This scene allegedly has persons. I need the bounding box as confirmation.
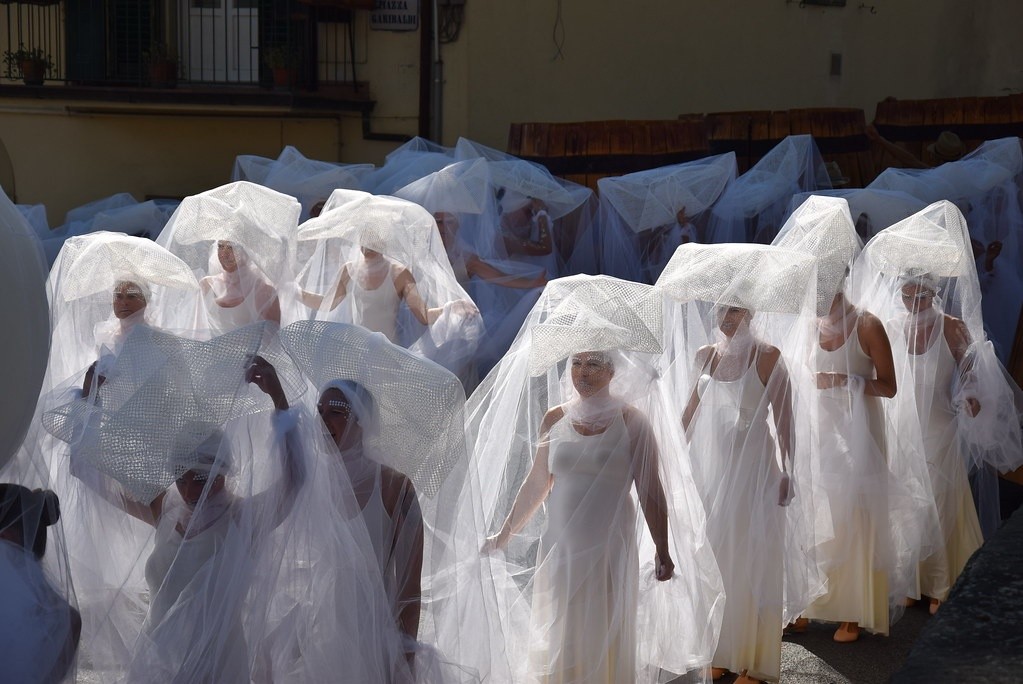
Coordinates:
[0,133,1023,684]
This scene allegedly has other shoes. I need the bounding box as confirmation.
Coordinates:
[698,667,729,679]
[833,622,859,642]
[731,674,761,684]
[928,598,941,614]
[785,617,809,633]
[895,596,916,607]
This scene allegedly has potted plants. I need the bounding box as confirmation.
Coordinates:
[2,42,55,85]
[142,40,186,88]
[265,44,306,86]
